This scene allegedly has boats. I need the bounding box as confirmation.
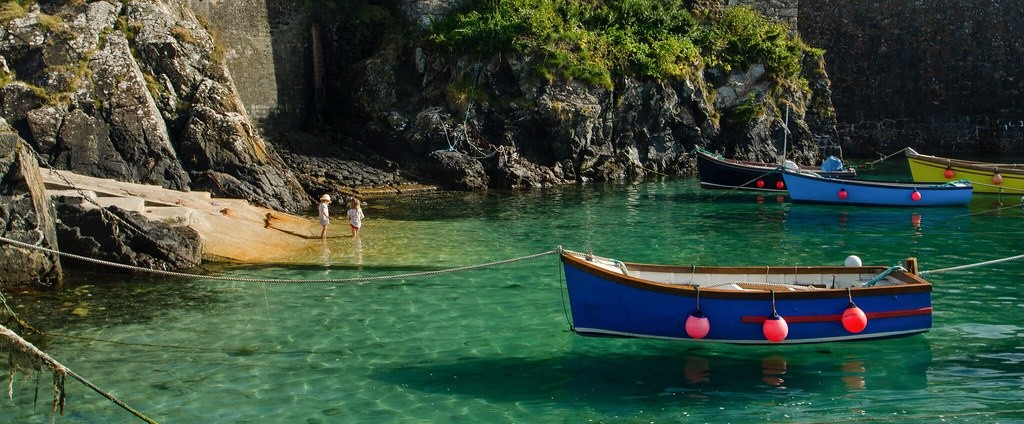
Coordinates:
[557,244,935,345]
[903,145,1024,196]
[779,160,973,208]
[694,102,857,207]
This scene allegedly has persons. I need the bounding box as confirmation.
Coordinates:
[319,194,332,239]
[347,198,365,239]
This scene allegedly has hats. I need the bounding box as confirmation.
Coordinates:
[320,194,331,203]
